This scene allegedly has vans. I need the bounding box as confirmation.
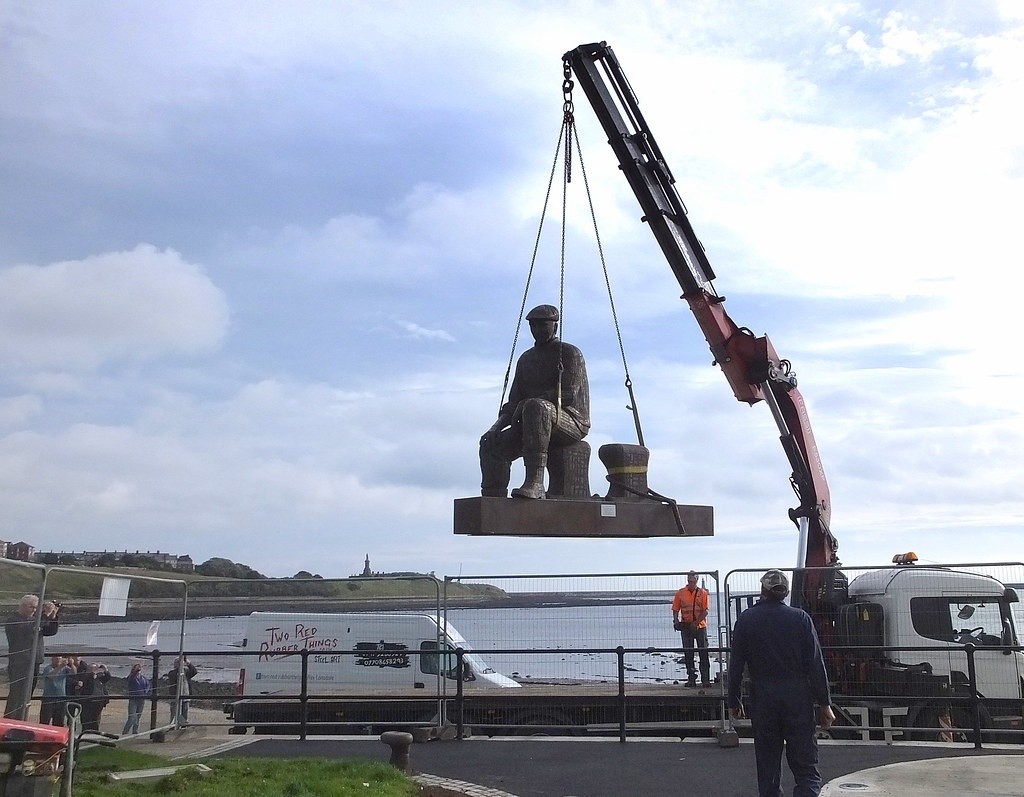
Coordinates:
[237,609,523,695]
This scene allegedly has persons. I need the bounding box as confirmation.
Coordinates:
[122,664,150,734]
[168,657,198,730]
[3,595,59,721]
[66,656,111,734]
[479,305,591,499]
[727,570,836,797]
[671,570,710,687]
[39,656,77,727]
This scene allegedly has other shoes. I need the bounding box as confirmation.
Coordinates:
[703,681,711,687]
[684,679,696,687]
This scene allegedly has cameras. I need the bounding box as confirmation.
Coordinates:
[51,599,63,606]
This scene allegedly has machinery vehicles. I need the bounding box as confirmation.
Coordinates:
[221,40,1023,747]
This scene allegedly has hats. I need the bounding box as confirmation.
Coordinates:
[760,569,789,597]
